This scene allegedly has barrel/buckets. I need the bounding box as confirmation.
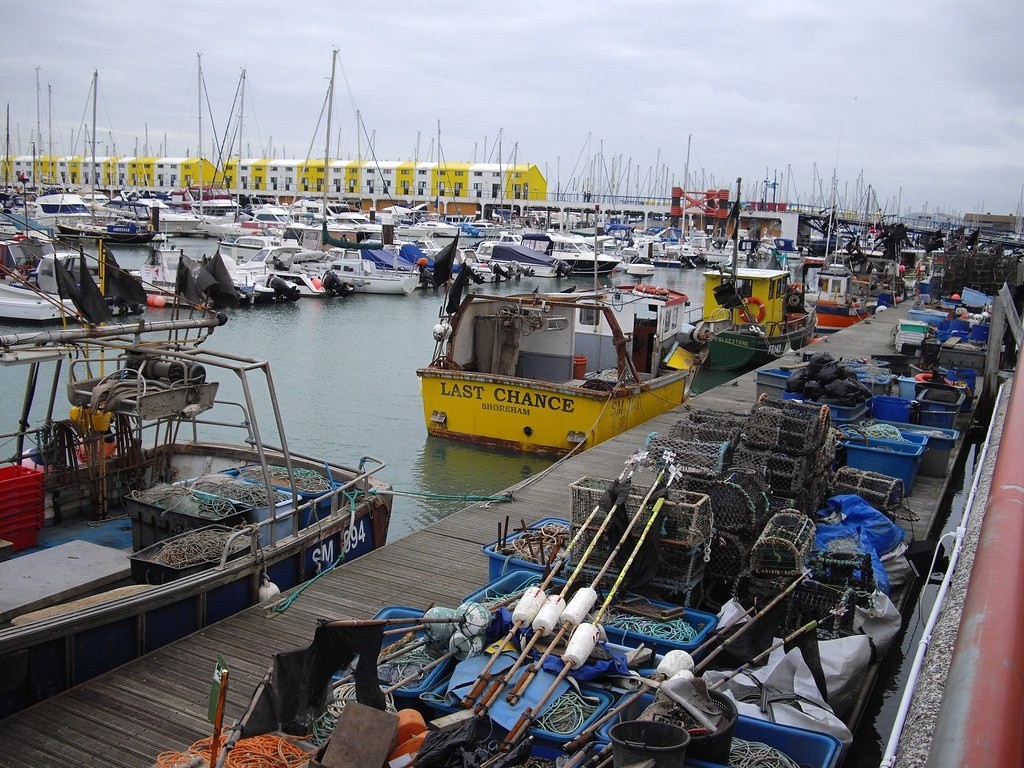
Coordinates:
[939,294,963,309]
[803,352,814,363]
[897,376,917,401]
[947,369,977,412]
[970,325,989,344]
[786,312,805,330]
[782,389,803,403]
[872,395,911,423]
[573,356,586,380]
[949,318,970,332]
[609,719,691,768]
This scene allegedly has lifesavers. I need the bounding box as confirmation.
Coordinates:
[793,283,802,293]
[914,372,953,387]
[636,284,670,295]
[739,297,766,324]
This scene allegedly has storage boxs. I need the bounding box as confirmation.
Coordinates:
[122,492,254,552]
[333,287,993,768]
[218,464,344,531]
[171,475,303,548]
[0,464,46,551]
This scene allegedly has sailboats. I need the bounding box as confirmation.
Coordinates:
[0,44,814,367]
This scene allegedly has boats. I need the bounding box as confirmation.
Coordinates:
[0,214,395,721]
[415,228,733,461]
[684,177,818,373]
[790,177,946,333]
